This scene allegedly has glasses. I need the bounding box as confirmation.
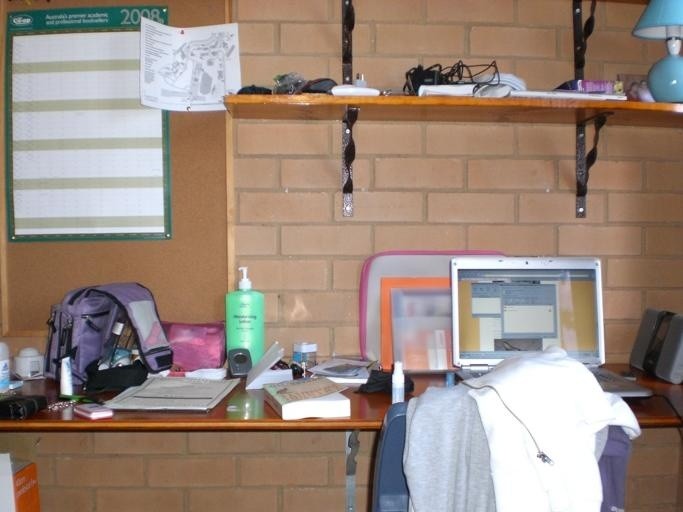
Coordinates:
[301,77,335,93]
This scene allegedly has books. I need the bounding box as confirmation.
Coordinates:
[266,373,356,421]
[311,358,376,385]
[103,375,240,412]
[245,341,293,390]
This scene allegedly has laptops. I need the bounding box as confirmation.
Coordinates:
[451,256,653,398]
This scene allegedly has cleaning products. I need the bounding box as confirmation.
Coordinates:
[391,360,405,403]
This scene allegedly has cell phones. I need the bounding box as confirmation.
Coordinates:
[74,404,113,420]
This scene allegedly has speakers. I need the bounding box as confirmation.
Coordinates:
[655,313,683,385]
[629,308,674,371]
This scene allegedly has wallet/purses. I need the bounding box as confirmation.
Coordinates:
[1,395,48,420]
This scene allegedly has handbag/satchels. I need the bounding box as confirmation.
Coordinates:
[167,321,226,370]
[44,286,126,387]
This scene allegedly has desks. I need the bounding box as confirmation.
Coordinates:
[0,363,681,512]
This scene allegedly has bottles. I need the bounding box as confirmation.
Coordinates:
[0,341,12,394]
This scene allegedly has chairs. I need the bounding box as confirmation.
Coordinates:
[373,401,631,512]
